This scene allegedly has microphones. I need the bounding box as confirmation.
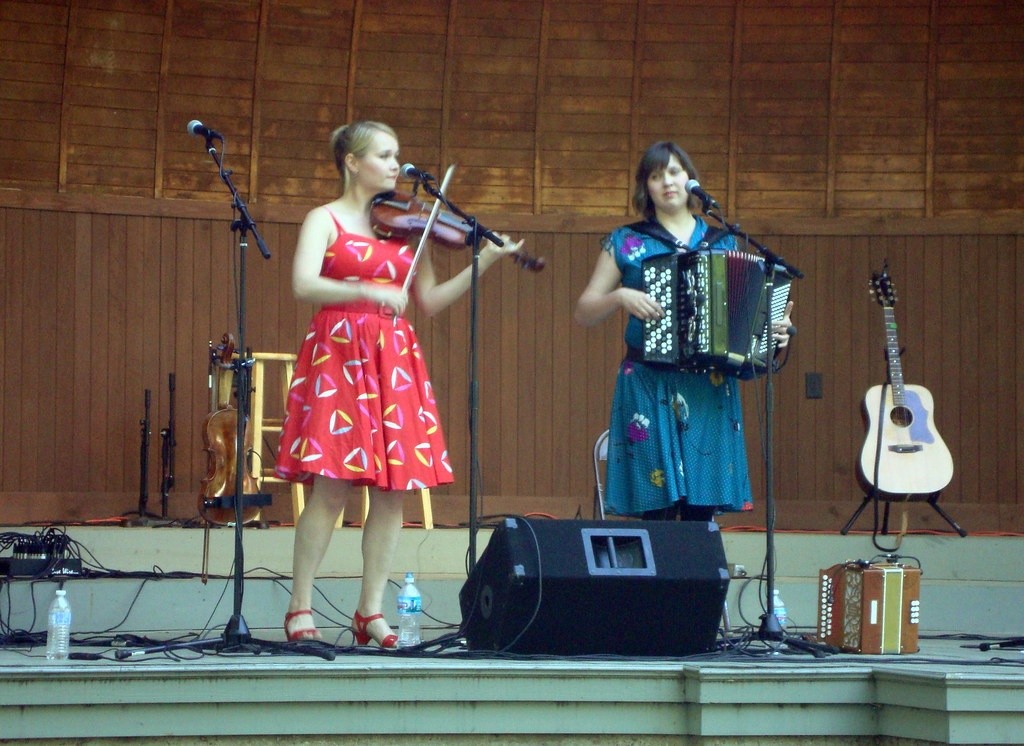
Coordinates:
[187,119,223,139]
[400,163,435,180]
[684,180,720,210]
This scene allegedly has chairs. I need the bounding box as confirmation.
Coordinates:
[593,429,731,634]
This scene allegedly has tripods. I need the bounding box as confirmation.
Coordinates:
[841,345,967,538]
[114,136,337,662]
[701,204,844,658]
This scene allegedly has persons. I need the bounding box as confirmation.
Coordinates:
[575,141,794,523]
[274,122,527,650]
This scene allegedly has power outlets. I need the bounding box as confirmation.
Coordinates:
[806,373,823,399]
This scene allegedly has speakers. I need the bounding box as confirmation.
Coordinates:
[459,520,731,660]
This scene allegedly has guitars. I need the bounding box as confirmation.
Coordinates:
[856,255,954,494]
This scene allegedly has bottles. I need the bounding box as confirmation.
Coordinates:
[765,590,786,655]
[46,591,71,660]
[398,574,422,649]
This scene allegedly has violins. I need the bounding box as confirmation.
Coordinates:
[199,331,261,526]
[371,191,545,273]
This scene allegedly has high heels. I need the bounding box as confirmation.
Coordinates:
[284,609,321,642]
[352,610,398,648]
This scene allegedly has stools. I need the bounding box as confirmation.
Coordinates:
[217,352,433,530]
